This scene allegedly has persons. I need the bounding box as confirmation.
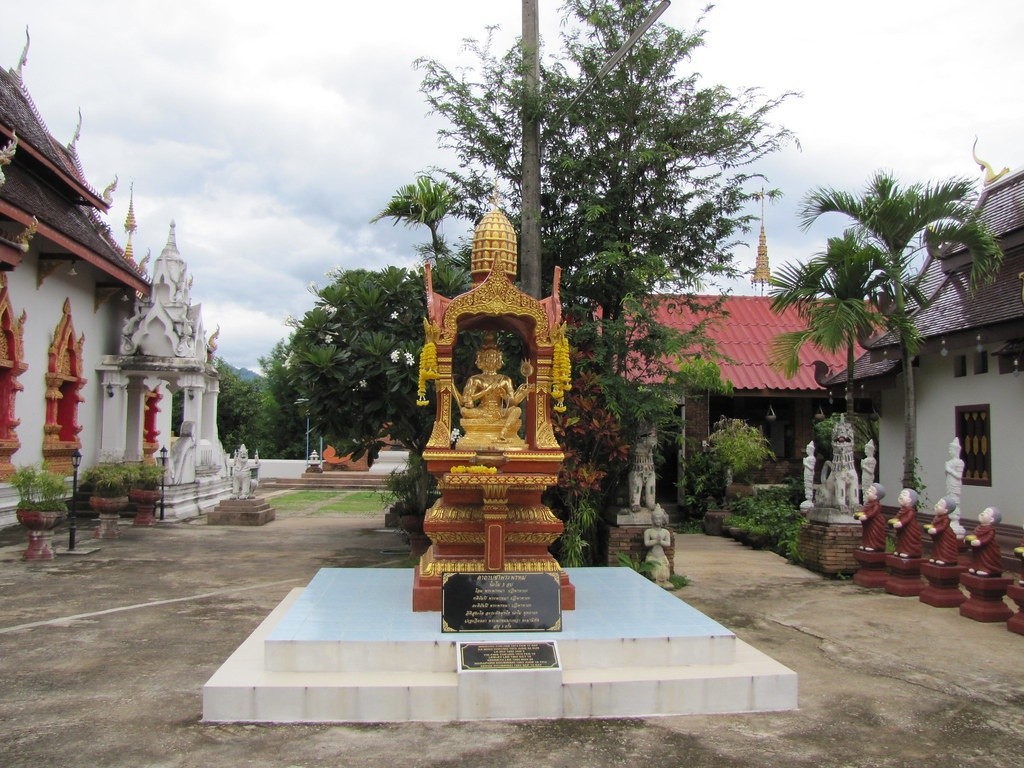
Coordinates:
[928,496,959,564]
[644,503,670,582]
[893,488,922,557]
[964,507,1003,575]
[452,335,535,441]
[628,427,657,512]
[803,440,816,500]
[853,483,886,550]
[945,437,964,523]
[860,438,876,505]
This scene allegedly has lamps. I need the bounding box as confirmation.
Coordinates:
[187,385,195,400]
[160,446,168,459]
[120,288,130,303]
[106,382,113,397]
[72,448,81,466]
[67,260,78,276]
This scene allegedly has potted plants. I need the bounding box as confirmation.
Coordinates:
[2,456,76,565]
[79,449,140,541]
[127,455,167,526]
[702,502,771,548]
[379,461,434,557]
